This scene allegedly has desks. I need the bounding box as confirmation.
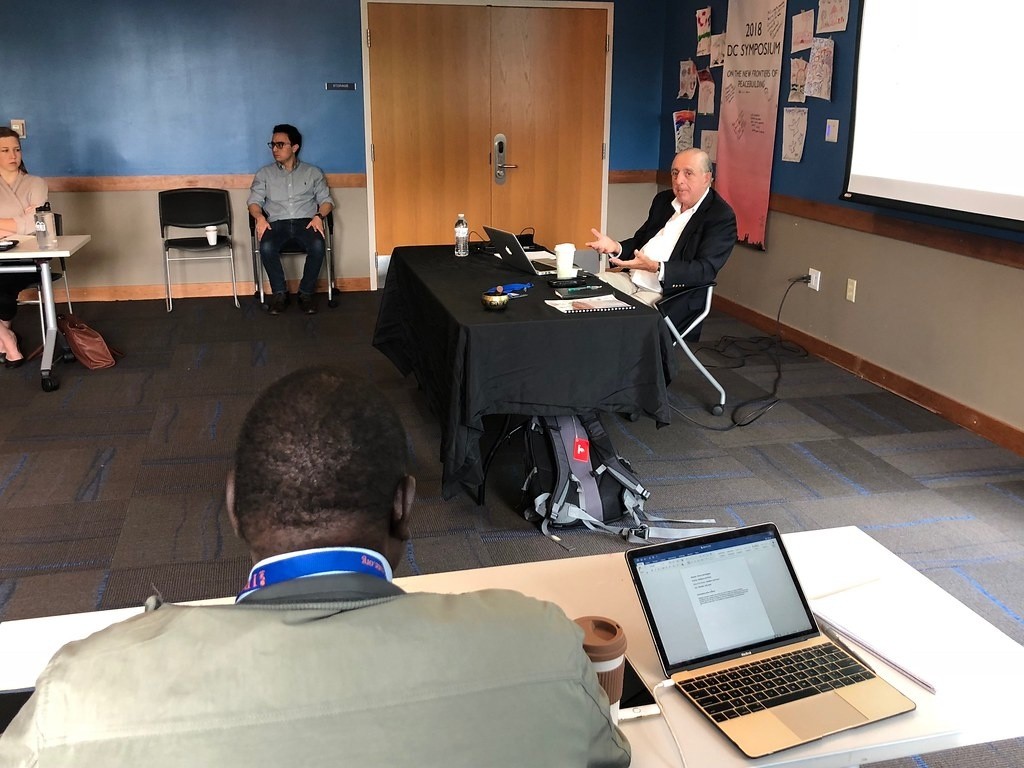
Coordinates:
[372,240,676,506]
[0,524,1024,767]
[0,235,93,392]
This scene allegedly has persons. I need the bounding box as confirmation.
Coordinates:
[248,124,336,317]
[0,367,632,768]
[0,126,49,366]
[584,147,737,329]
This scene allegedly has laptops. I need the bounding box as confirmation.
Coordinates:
[483,226,582,276]
[624,522,917,758]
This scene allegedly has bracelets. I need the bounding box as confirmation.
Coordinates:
[314,212,325,220]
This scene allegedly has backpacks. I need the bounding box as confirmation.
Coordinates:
[496,412,651,550]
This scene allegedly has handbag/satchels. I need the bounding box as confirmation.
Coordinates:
[57,314,126,370]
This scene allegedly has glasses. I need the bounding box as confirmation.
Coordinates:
[267,142,291,149]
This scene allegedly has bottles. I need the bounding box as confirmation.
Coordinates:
[34,202,58,251]
[454,213,470,256]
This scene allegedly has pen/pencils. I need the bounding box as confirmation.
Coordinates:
[567,286,602,292]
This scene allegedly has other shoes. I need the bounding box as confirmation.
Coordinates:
[294,291,316,315]
[0,353,6,364]
[268,292,288,315]
[4,330,25,370]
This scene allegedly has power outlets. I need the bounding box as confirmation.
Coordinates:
[808,267,820,291]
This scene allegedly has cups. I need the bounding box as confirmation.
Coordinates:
[572,616,628,727]
[555,243,576,280]
[205,225,217,245]
[481,292,509,311]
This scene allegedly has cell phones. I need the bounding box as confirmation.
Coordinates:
[619,654,662,721]
[547,279,586,288]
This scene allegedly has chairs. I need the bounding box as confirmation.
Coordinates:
[653,281,726,415]
[25,214,74,348]
[249,204,341,312]
[158,189,241,313]
